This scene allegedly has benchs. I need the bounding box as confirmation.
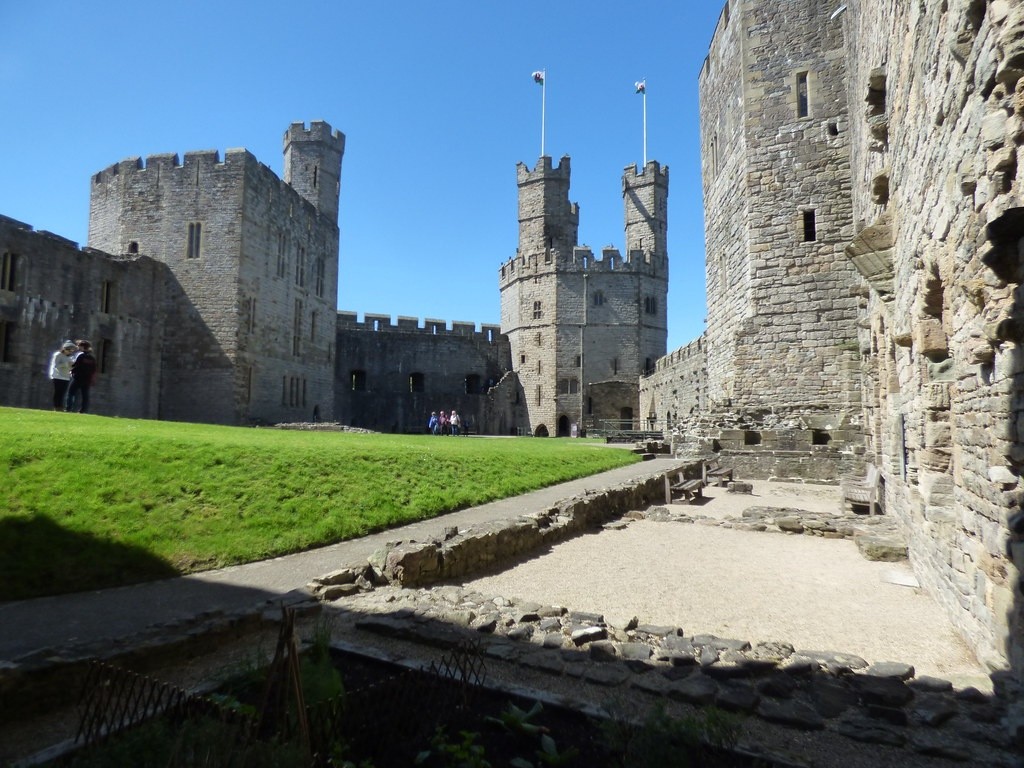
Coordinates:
[840,463,882,516]
[664,465,702,504]
[702,455,732,486]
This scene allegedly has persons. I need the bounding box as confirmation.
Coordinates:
[63,339,96,413]
[429,410,470,437]
[49,340,76,411]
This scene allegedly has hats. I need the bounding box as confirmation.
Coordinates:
[63,340,75,350]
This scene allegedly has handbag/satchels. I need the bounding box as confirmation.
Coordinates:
[458,423,461,428]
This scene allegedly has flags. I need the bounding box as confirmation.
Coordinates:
[635,81,645,94]
[532,71,544,86]
